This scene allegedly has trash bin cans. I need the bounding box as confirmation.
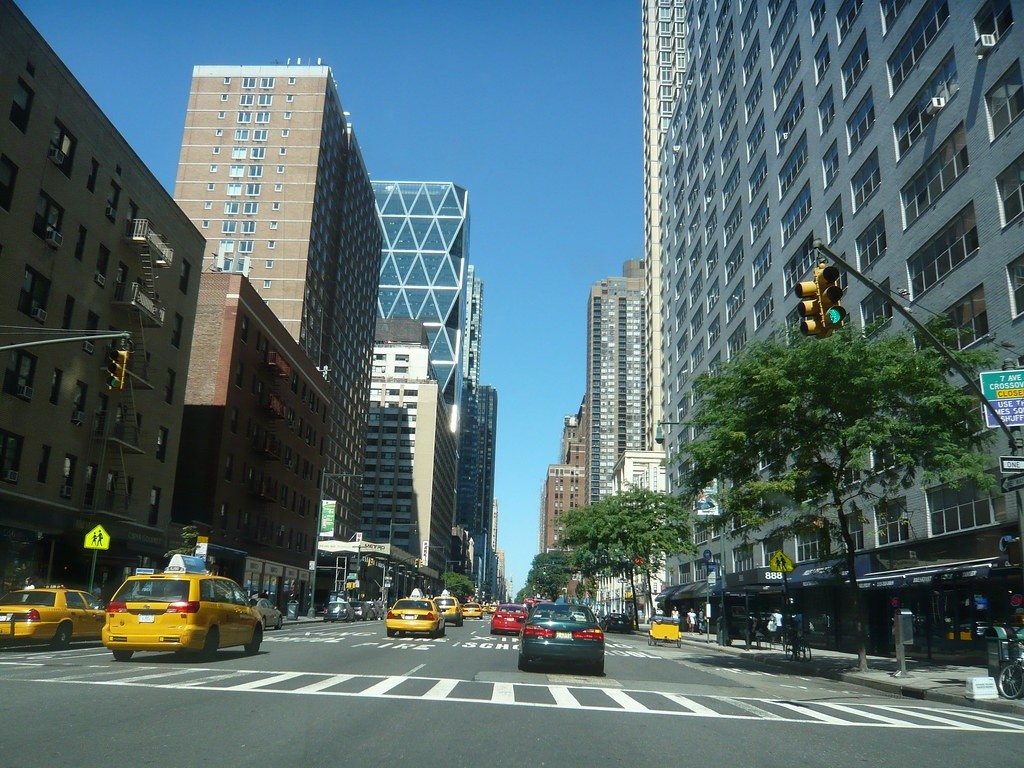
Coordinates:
[287,600,300,621]
[717,616,729,642]
[895,608,914,645]
[985,626,1024,696]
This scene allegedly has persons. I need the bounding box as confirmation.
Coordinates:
[377,597,385,617]
[92,580,102,599]
[288,593,296,600]
[671,606,680,620]
[595,609,601,623]
[766,608,784,645]
[696,611,706,634]
[687,607,697,633]
[268,591,276,605]
[260,589,268,598]
[20,576,35,602]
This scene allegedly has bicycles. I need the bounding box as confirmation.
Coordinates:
[997,637,1024,700]
[784,624,811,662]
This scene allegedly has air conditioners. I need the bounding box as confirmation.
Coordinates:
[927,96,944,114]
[975,34,994,54]
[61,486,72,498]
[781,132,788,143]
[672,145,679,154]
[106,207,118,221]
[47,149,65,164]
[3,470,18,482]
[83,341,94,353]
[72,411,86,422]
[31,307,47,322]
[17,385,32,399]
[45,230,64,246]
[94,274,106,286]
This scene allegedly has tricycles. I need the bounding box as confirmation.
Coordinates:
[647,615,682,648]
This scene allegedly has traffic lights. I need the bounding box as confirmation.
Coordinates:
[794,280,829,339]
[814,265,847,330]
[419,559,424,569]
[106,350,130,389]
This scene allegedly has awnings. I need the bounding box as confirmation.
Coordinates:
[856,555,1001,590]
[711,581,753,597]
[655,577,720,602]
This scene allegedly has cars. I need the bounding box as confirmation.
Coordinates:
[481,598,546,614]
[101,553,265,663]
[323,600,385,623]
[461,603,483,620]
[385,588,445,639]
[515,601,605,676]
[598,612,632,635]
[0,584,109,648]
[432,589,464,627]
[490,603,528,636]
[249,597,283,631]
